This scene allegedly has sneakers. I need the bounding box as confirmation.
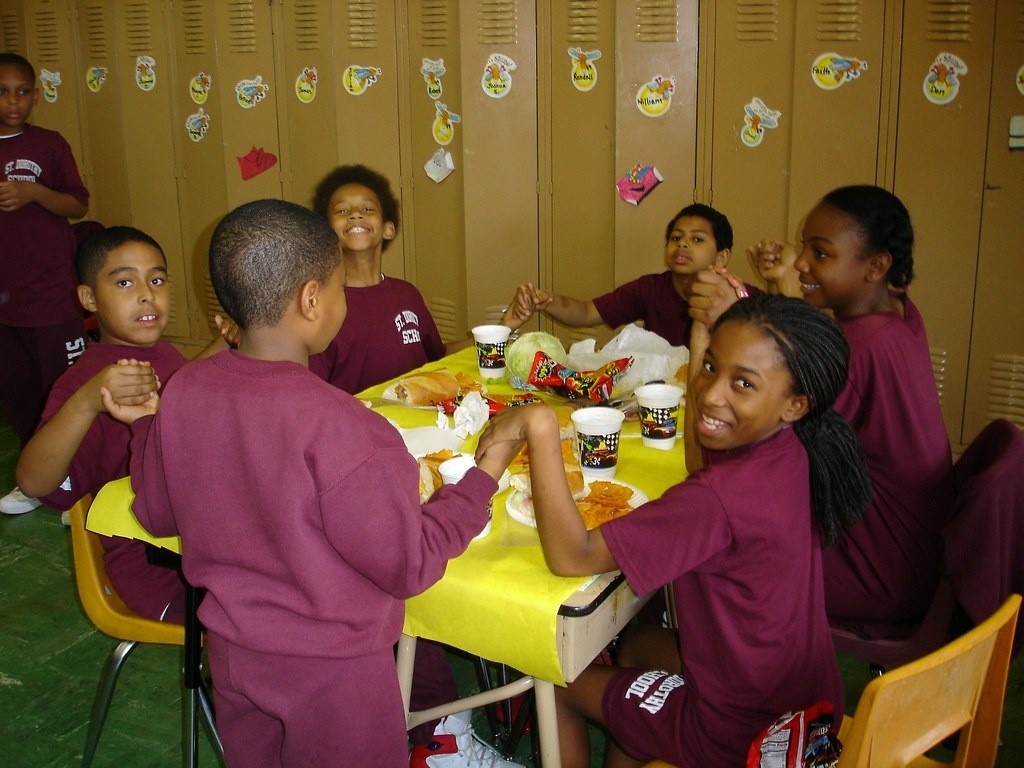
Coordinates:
[0,486,42,514]
[410,709,525,768]
[61,510,71,527]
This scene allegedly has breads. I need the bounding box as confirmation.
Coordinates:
[417,448,463,502]
[674,363,688,384]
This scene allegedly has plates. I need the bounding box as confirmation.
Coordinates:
[504,474,650,529]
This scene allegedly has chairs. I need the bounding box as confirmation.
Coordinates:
[61,492,227,768]
[817,416,1024,768]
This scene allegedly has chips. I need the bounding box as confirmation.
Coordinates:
[577,480,636,530]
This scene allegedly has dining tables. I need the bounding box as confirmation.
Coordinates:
[86,332,691,768]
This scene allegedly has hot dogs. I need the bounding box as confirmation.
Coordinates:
[395,368,483,405]
[509,463,585,498]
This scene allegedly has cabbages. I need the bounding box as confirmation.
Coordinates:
[504,331,569,387]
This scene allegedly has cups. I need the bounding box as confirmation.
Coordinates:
[472,325,511,379]
[635,385,684,451]
[571,407,626,478]
[438,456,493,540]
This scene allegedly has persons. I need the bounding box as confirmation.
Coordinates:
[510,202,766,348]
[686,184,954,625]
[16,224,455,747]
[0,53,90,515]
[99,199,525,768]
[221,166,553,395]
[476,293,844,768]
